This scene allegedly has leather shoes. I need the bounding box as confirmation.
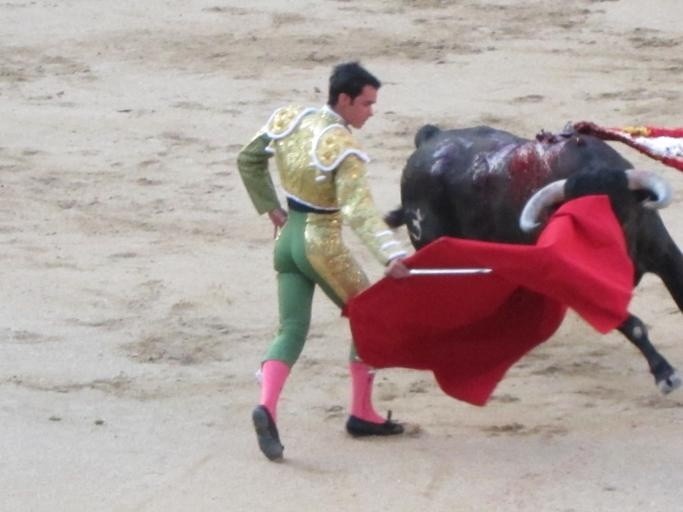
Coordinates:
[346,414,404,436]
[252,405,283,464]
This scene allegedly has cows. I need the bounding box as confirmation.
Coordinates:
[382,123,682,395]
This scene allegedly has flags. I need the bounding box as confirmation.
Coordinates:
[333,193,634,408]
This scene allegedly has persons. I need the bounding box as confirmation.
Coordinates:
[235,60,406,462]
